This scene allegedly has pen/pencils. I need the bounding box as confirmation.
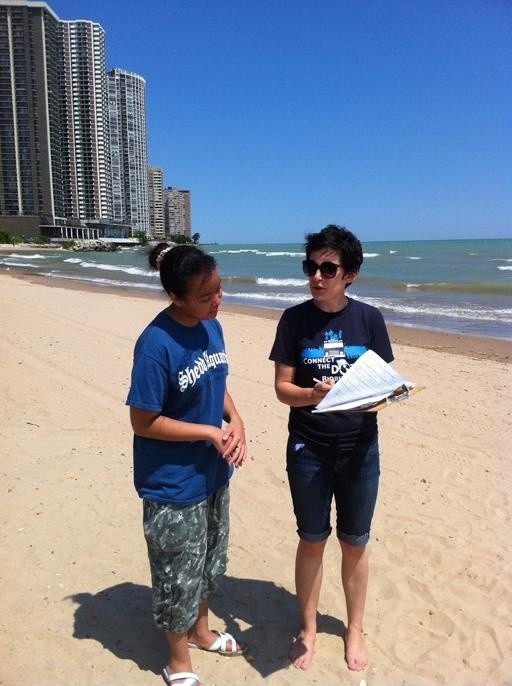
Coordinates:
[313,378,333,389]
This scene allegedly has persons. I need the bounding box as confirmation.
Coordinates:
[126,243,247,686]
[268,224,395,672]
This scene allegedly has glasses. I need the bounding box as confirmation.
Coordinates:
[302,260,344,279]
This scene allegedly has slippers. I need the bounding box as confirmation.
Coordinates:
[187,629,249,657]
[163,666,201,685]
